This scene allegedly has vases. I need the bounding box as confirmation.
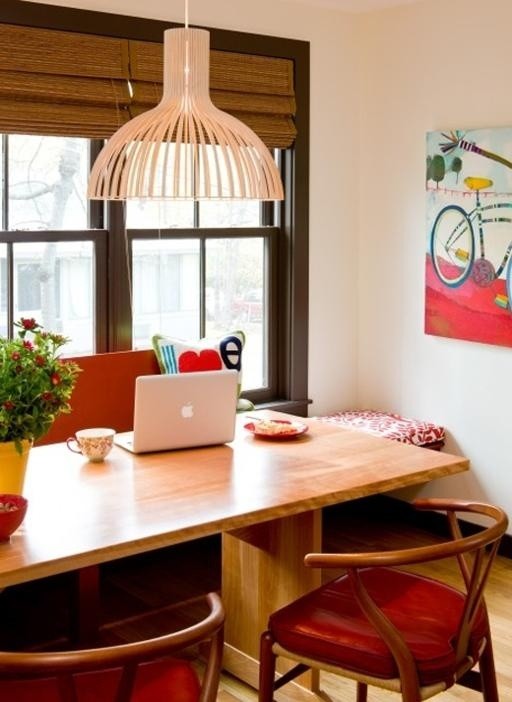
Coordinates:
[0,436,33,498]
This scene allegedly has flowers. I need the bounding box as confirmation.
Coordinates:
[0,318,86,445]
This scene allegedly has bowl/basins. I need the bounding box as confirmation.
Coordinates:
[0,493,28,544]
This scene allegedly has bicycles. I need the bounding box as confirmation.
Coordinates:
[431,177,512,311]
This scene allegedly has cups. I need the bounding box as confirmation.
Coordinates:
[67,429,115,464]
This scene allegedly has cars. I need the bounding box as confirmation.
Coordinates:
[228,294,262,320]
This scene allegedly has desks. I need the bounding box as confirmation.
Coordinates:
[307,409,447,451]
[2,408,470,702]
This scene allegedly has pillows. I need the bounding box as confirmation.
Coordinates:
[151,329,256,412]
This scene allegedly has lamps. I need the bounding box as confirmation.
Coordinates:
[86,0,288,202]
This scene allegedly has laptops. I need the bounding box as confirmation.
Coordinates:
[111,369,238,453]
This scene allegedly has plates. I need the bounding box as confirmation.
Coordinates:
[244,419,313,441]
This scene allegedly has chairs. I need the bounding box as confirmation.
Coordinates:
[0,591,225,700]
[255,496,509,702]
[36,348,165,448]
